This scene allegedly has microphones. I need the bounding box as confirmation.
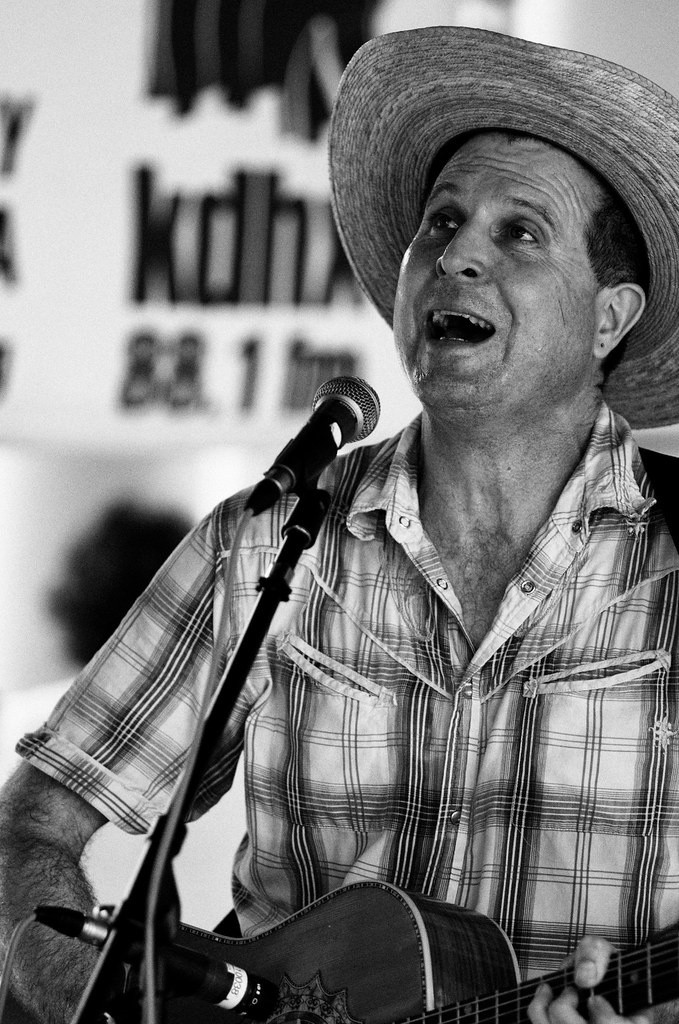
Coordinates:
[244,376,381,517]
[33,905,277,1020]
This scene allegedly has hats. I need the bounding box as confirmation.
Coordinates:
[326,26,679,429]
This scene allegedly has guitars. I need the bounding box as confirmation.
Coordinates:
[163,880,679,1024]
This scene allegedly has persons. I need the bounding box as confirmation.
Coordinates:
[0,26,679,1024]
[1,502,244,933]
